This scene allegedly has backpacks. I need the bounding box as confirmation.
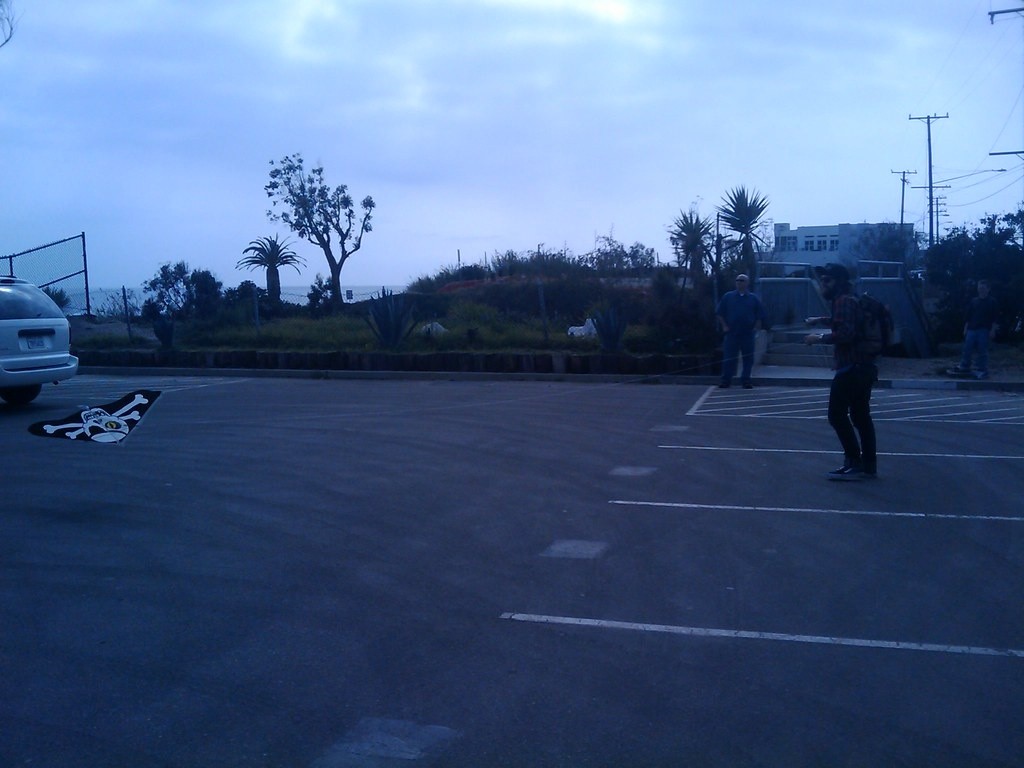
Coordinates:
[854,293,893,355]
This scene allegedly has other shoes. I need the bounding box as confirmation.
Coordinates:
[948,368,969,374]
[740,379,752,388]
[718,384,730,388]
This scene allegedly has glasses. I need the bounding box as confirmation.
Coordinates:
[735,279,746,282]
[818,277,837,283]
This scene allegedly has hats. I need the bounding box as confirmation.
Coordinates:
[815,263,849,283]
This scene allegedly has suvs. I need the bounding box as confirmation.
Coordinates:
[0,274,80,404]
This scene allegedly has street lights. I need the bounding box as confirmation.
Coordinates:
[928,169,1007,249]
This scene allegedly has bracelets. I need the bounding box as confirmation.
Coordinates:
[819,334,823,343]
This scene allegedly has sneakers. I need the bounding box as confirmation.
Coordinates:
[859,465,877,477]
[825,465,864,481]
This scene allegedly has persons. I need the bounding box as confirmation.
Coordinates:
[715,274,763,388]
[947,278,998,379]
[802,263,883,480]
